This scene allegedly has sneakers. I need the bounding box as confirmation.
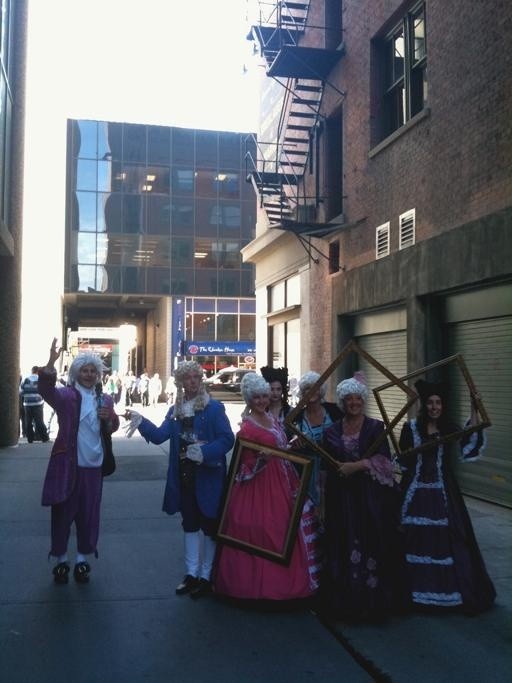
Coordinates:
[190,577,209,597]
[52,563,69,583]
[73,561,90,583]
[175,574,198,595]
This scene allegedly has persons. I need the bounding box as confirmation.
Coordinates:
[116,360,236,599]
[322,378,406,622]
[138,373,149,408]
[211,372,322,602]
[294,370,345,507]
[125,370,137,407]
[36,337,121,585]
[259,365,296,444]
[21,365,51,444]
[106,369,118,401]
[395,379,497,616]
[148,373,163,406]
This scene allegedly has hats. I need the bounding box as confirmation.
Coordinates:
[415,373,444,396]
[260,367,282,381]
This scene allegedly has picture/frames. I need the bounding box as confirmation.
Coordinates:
[212,435,315,567]
[372,352,492,462]
[282,341,418,482]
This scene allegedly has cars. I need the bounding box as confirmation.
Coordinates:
[202,367,256,402]
